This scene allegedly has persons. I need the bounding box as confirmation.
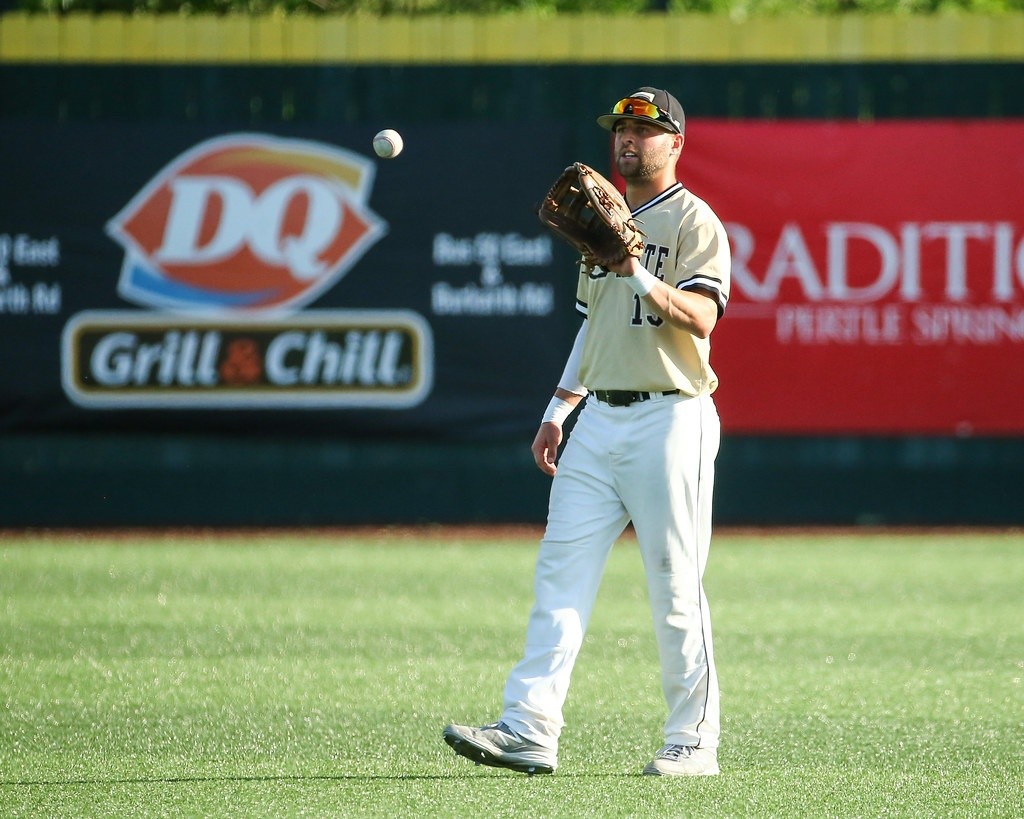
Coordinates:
[443,87,731,779]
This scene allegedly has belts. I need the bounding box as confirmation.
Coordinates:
[587,389,681,407]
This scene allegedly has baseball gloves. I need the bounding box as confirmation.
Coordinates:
[538,162,645,273]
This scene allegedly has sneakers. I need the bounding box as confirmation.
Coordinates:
[643,744,719,776]
[443,721,557,777]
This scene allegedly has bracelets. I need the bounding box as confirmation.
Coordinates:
[541,395,575,427]
[621,265,663,299]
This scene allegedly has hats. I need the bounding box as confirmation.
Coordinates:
[597,87,685,136]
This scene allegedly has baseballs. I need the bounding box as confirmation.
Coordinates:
[372,129,405,159]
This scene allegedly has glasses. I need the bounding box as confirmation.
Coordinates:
[611,98,680,134]
